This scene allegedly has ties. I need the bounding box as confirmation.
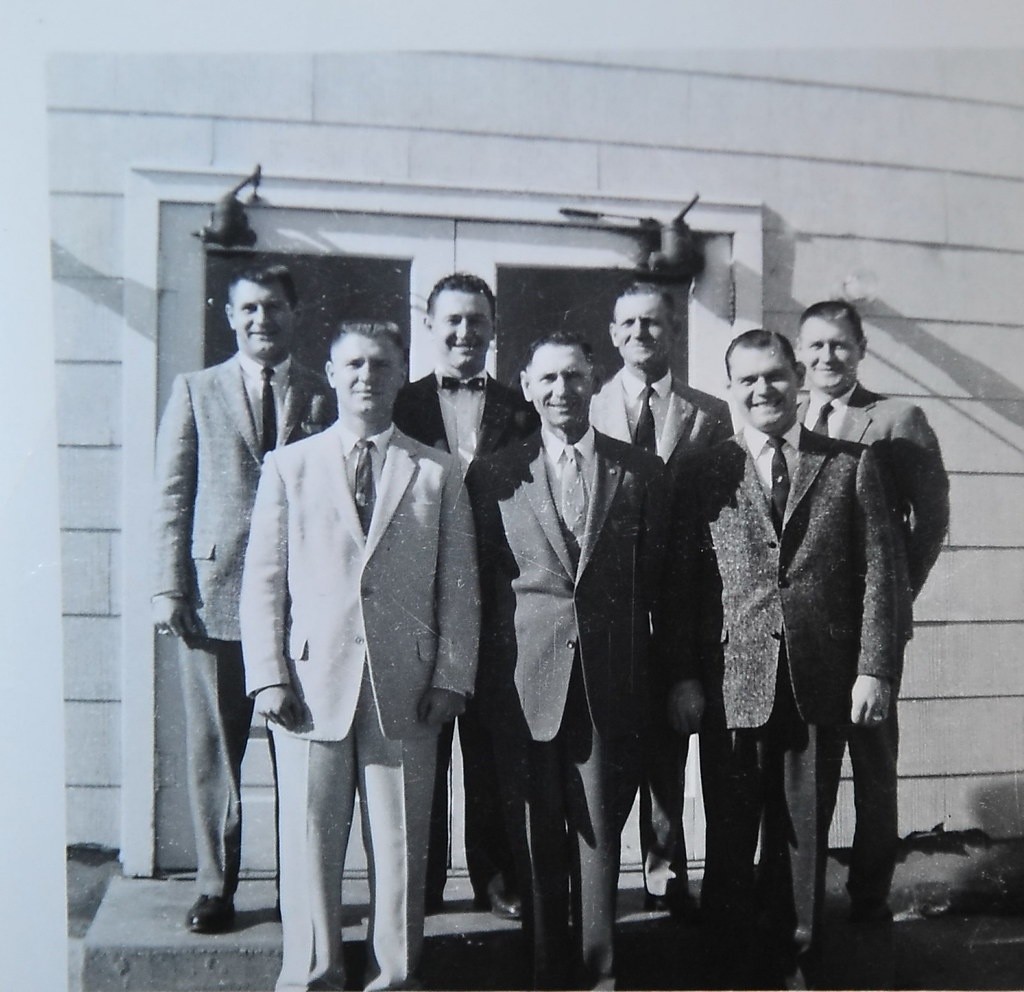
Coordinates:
[632,386,656,455]
[562,445,588,575]
[766,436,790,540]
[812,402,835,436]
[262,368,279,454]
[353,440,376,538]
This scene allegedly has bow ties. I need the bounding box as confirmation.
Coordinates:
[440,376,486,390]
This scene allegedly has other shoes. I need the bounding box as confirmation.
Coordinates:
[646,891,703,921]
[853,895,893,925]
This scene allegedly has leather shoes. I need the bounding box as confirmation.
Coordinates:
[187,893,238,931]
[474,884,522,918]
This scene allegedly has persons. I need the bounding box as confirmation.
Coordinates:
[794,302,950,944]
[461,331,678,991]
[393,274,542,918]
[150,261,339,931]
[239,320,481,992]
[676,333,900,991]
[589,283,735,910]
